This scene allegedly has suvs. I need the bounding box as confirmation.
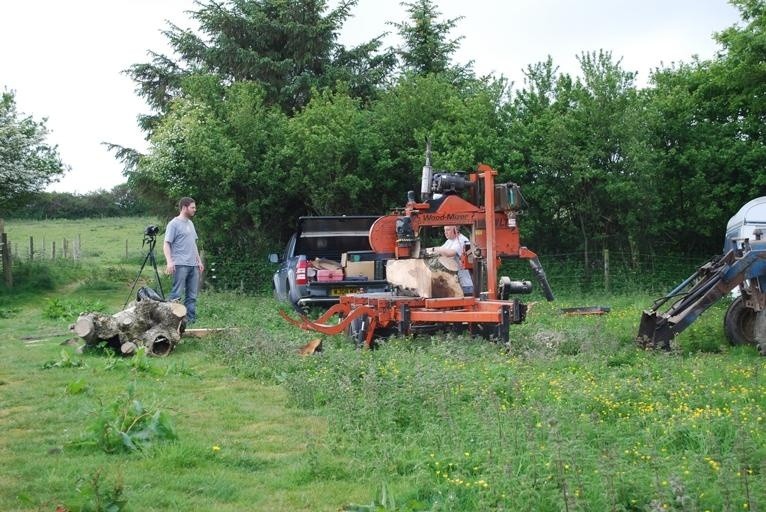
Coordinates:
[267,213,398,315]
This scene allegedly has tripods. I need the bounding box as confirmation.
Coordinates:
[123,238,170,311]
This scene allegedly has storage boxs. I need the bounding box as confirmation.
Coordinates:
[346,261,375,281]
[317,270,343,281]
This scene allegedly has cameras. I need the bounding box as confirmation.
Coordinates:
[145,226,158,236]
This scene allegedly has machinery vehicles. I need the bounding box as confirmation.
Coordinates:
[636,195,766,353]
[276,135,610,352]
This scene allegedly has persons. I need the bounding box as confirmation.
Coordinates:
[163,197,204,323]
[434,225,473,294]
